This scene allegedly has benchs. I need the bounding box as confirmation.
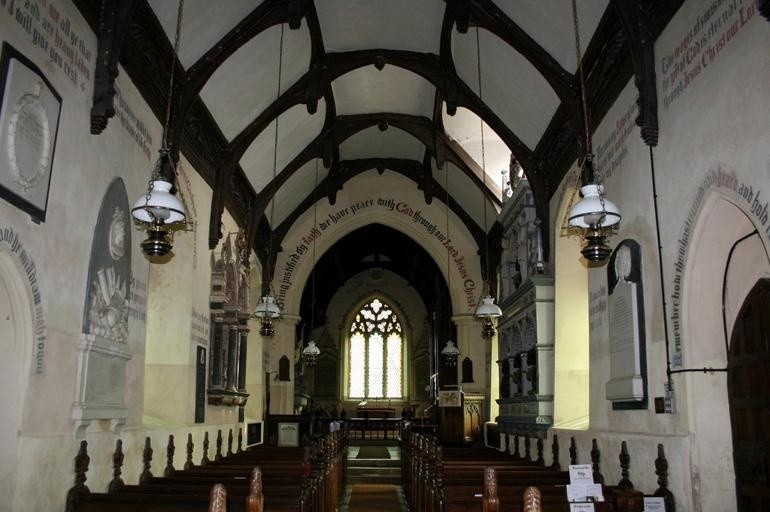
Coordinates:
[65,430,346,512]
[402,429,676,512]
